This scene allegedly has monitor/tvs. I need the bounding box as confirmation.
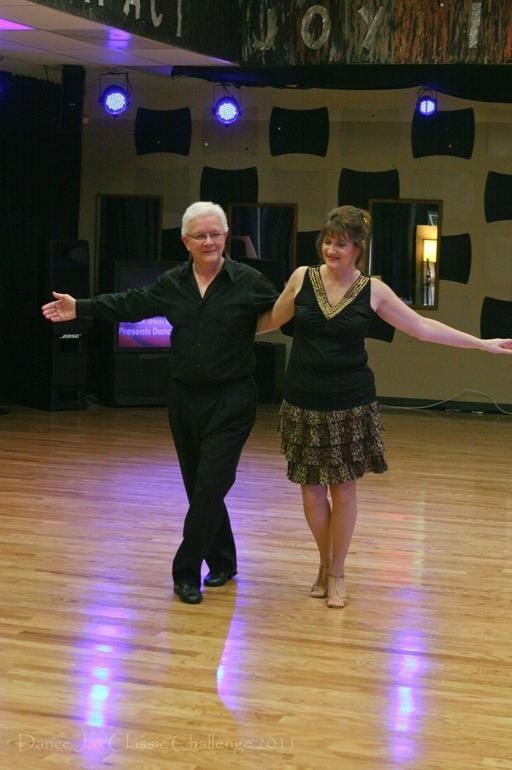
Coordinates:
[112,316,173,353]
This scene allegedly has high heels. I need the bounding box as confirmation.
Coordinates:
[310,566,329,598]
[326,574,346,608]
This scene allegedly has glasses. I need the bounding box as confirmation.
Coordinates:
[187,231,226,240]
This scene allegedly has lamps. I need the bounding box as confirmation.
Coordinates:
[96,71,131,117]
[415,223,438,264]
[414,85,439,117]
[211,84,242,126]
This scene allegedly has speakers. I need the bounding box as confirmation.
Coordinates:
[36,235,90,411]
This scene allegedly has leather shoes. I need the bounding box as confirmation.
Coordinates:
[174,583,202,603]
[205,567,237,586]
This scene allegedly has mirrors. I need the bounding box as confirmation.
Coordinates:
[225,202,298,295]
[364,197,442,311]
[94,191,163,298]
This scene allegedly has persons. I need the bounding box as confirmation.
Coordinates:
[256,205,512,609]
[42,201,295,603]
